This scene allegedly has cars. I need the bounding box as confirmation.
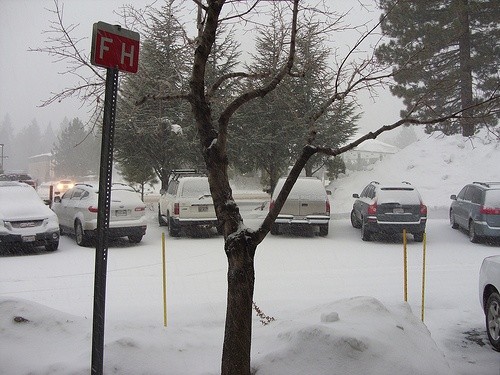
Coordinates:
[478,254,500,352]
[0,180,60,253]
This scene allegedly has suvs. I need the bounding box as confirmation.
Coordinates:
[50,182,148,246]
[157,171,222,237]
[350,180,428,242]
[450,181,500,243]
[267,175,331,235]
